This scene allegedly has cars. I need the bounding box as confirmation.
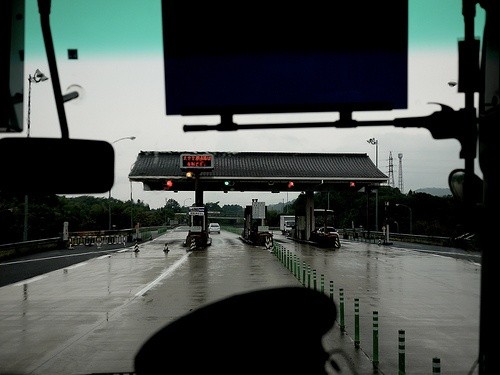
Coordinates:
[207,222,221,234]
[317,226,339,239]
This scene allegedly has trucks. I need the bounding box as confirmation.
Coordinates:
[279,215,295,235]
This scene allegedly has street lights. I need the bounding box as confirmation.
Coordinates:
[109,137,136,230]
[22,68,49,242]
[395,204,413,234]
[184,198,192,207]
